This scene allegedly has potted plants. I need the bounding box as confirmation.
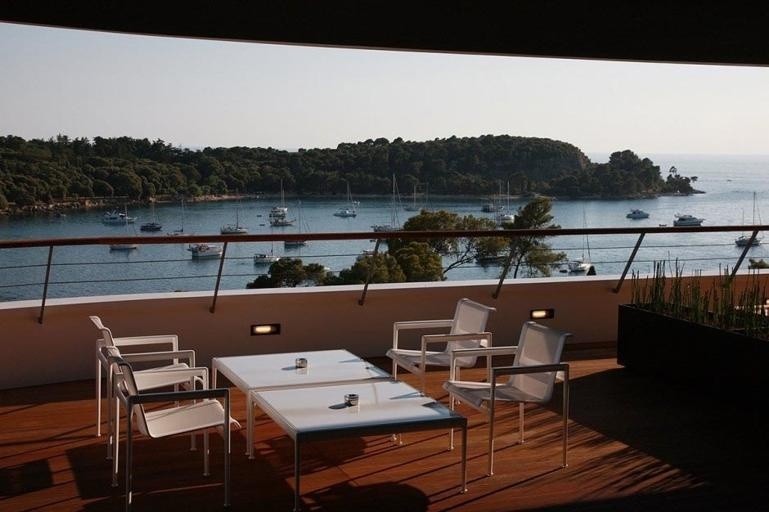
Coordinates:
[616,253,769,408]
[723,281,769,444]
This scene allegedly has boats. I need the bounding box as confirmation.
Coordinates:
[482,204,505,212]
[495,215,518,225]
[164,230,191,242]
[403,204,420,211]
[268,206,290,218]
[191,244,223,259]
[733,235,764,248]
[269,218,295,226]
[105,210,137,225]
[286,238,308,248]
[673,213,705,228]
[221,226,248,237]
[111,241,138,252]
[626,208,651,219]
[334,207,357,217]
[254,251,283,265]
[141,221,161,231]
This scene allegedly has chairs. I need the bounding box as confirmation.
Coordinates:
[385,298,499,406]
[441,322,574,477]
[87,314,241,511]
